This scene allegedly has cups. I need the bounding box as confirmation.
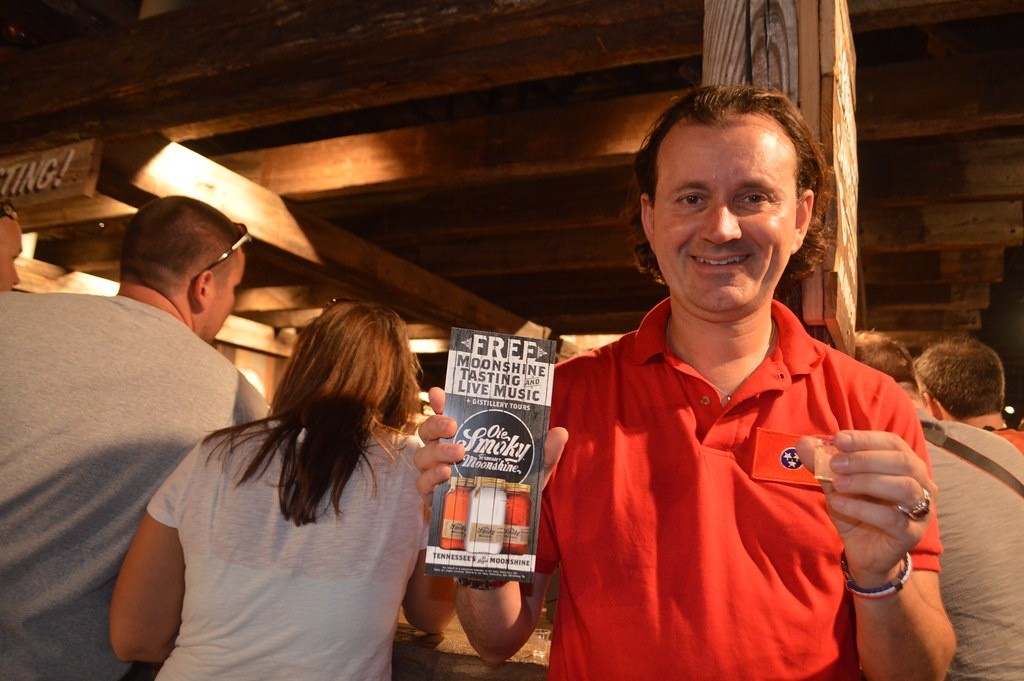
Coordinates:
[530,629,550,656]
[812,434,844,483]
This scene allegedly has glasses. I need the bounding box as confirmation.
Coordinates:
[324,296,400,319]
[190,222,252,284]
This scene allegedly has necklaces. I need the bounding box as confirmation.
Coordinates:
[666,313,775,400]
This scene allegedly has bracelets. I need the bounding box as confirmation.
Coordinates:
[455,576,510,589]
[840,552,912,598]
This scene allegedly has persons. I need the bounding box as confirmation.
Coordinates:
[0,196,268,681]
[856,327,1024,681]
[416,85,958,681]
[106,301,454,681]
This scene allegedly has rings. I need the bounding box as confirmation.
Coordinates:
[898,488,931,521]
